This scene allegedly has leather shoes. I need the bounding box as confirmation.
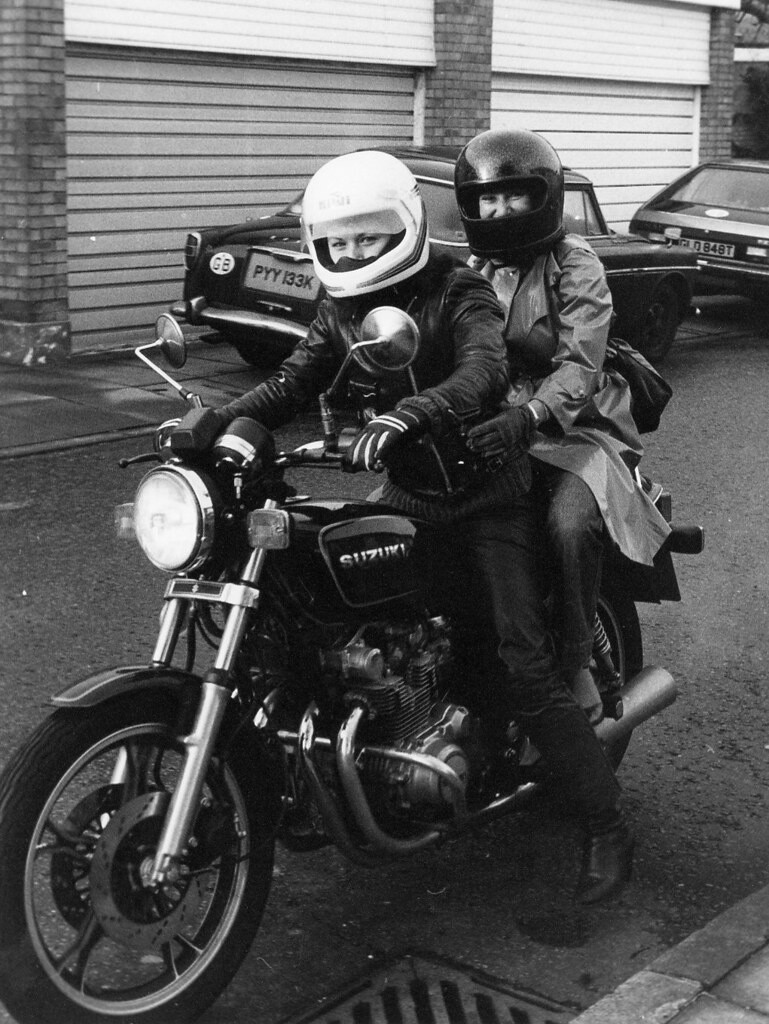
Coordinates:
[571,812,635,907]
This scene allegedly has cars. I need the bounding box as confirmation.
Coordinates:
[180,142,702,379]
[626,154,769,303]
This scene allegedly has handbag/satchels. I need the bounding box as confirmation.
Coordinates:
[605,338,673,434]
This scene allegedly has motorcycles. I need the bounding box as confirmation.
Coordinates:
[0,301,706,1024]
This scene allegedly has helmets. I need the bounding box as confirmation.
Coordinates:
[453,128,564,258]
[299,150,430,297]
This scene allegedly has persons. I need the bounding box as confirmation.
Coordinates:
[151,148,652,903]
[453,130,674,723]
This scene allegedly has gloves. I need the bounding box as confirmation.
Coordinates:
[466,400,536,459]
[341,404,432,474]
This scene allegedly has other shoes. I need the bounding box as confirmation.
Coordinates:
[568,666,605,726]
